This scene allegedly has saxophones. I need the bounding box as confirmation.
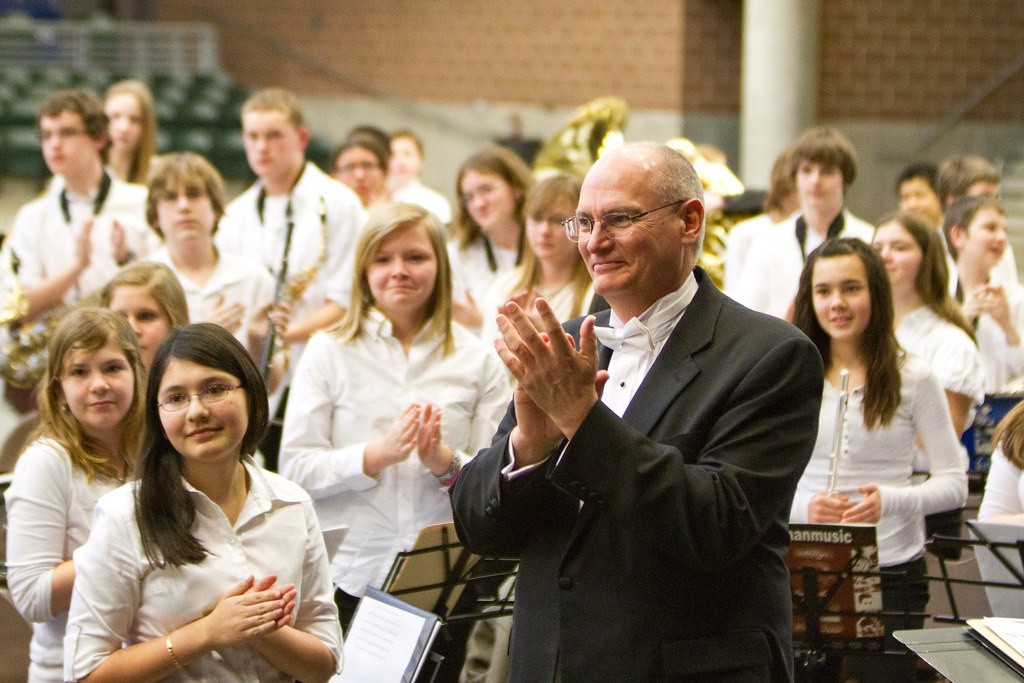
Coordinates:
[291,194,332,298]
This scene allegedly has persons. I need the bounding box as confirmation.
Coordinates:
[0,79,164,337]
[976,399,1024,519]
[224,86,453,478]
[64,323,344,683]
[277,201,514,683]
[725,125,876,323]
[449,145,535,348]
[481,173,611,389]
[143,152,289,461]
[447,140,825,683]
[897,153,1024,475]
[872,212,982,560]
[3,305,150,683]
[793,237,970,644]
[100,260,189,370]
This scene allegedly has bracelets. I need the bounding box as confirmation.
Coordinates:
[166,635,185,670]
[433,446,461,486]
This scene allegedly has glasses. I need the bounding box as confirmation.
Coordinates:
[561,199,684,243]
[156,383,243,412]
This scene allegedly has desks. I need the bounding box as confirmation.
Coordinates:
[888,626,1024,683]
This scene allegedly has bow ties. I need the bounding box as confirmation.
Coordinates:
[593,282,698,351]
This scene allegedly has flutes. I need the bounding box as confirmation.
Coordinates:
[260,218,294,382]
